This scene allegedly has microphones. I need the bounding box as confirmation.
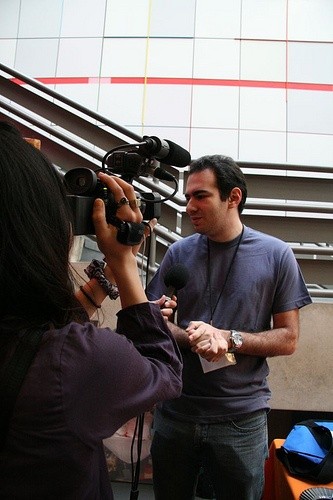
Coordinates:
[160,263,190,311]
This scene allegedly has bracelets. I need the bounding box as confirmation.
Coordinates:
[84,259,120,300]
[79,285,101,308]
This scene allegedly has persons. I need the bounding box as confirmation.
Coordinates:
[0,120,185,500]
[149,294,178,324]
[144,155,313,500]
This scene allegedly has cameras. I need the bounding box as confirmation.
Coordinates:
[64,136,191,235]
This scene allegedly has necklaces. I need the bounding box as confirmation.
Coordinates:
[207,223,245,325]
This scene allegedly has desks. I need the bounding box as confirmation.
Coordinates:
[265,437,333,500]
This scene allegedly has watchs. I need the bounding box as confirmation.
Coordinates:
[227,329,244,354]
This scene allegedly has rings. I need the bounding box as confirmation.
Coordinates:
[116,197,130,209]
[129,199,141,208]
